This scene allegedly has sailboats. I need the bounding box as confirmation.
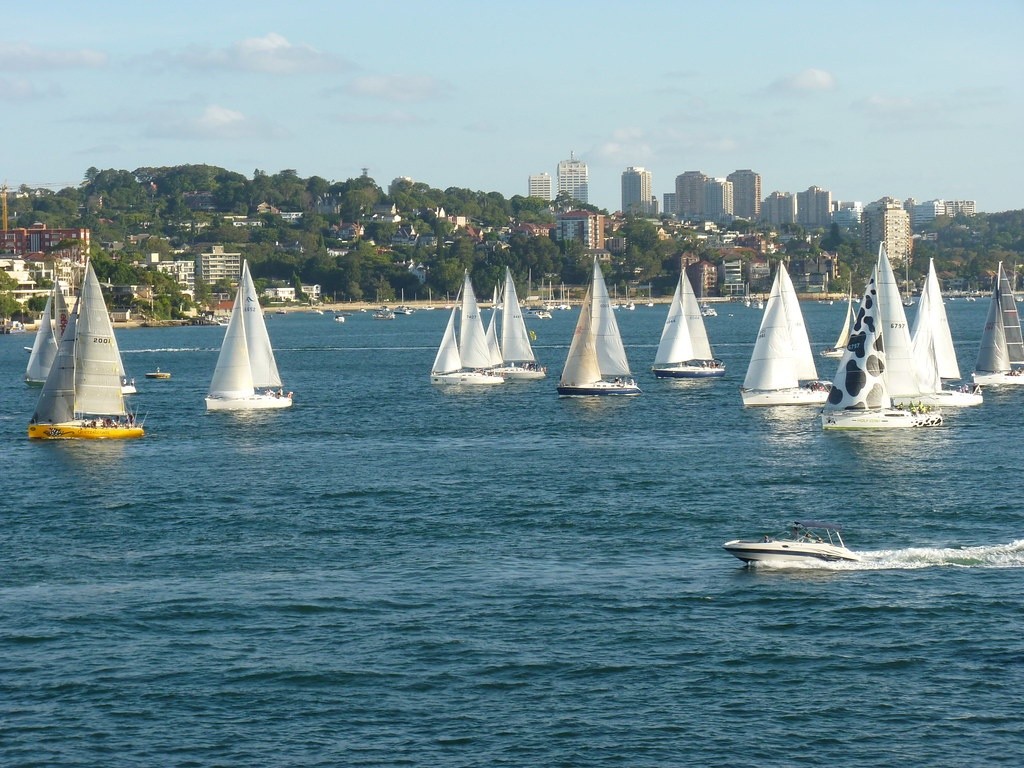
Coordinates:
[24,276,70,386]
[902,247,915,307]
[971,261,1024,385]
[698,273,717,316]
[819,286,856,358]
[539,278,571,310]
[445,291,452,309]
[430,274,504,385]
[892,258,984,407]
[205,259,293,410]
[744,281,751,307]
[29,256,144,440]
[612,283,636,310]
[1012,261,1024,302]
[645,282,654,307]
[556,256,642,397]
[820,242,943,429]
[740,260,833,405]
[651,266,726,378]
[425,288,435,310]
[484,267,546,380]
[112,329,136,393]
[842,271,860,303]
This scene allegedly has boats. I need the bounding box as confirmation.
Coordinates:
[965,297,976,302]
[0,321,26,334]
[949,297,954,301]
[522,311,552,320]
[393,306,414,314]
[721,521,861,565]
[372,312,396,320]
[751,299,764,310]
[276,306,367,324]
